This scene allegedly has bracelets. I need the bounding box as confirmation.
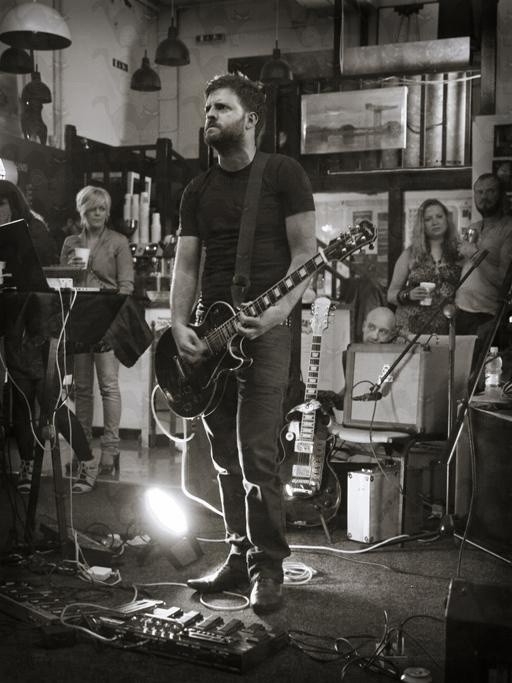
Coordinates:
[395,289,412,304]
[468,251,481,260]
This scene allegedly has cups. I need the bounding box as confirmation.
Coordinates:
[484,346,503,388]
[418,282,436,305]
[123,192,162,244]
[74,247,92,270]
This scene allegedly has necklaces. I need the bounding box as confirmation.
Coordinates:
[426,245,450,268]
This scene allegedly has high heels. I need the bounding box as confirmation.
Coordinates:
[100,447,120,474]
[17,459,35,496]
[73,456,100,493]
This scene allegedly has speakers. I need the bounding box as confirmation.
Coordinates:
[454,402,511,566]
[443,576,511,683]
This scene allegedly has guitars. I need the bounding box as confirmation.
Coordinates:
[152,220,378,421]
[275,293,341,531]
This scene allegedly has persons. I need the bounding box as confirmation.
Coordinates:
[451,173,510,341]
[0,179,101,494]
[56,183,136,476]
[386,198,461,335]
[165,72,321,616]
[360,307,402,347]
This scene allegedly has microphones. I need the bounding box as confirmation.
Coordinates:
[475,248,490,268]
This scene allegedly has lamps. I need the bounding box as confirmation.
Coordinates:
[258,2,293,82]
[0,0,73,104]
[129,1,191,91]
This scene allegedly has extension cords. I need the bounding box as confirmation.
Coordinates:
[103,532,151,549]
[376,641,410,670]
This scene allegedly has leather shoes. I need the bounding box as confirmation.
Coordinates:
[186,562,247,593]
[249,576,284,612]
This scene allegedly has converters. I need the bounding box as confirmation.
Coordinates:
[88,565,112,581]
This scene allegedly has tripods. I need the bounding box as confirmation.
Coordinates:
[357,299,511,568]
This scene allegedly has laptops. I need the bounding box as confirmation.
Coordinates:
[1,217,121,294]
